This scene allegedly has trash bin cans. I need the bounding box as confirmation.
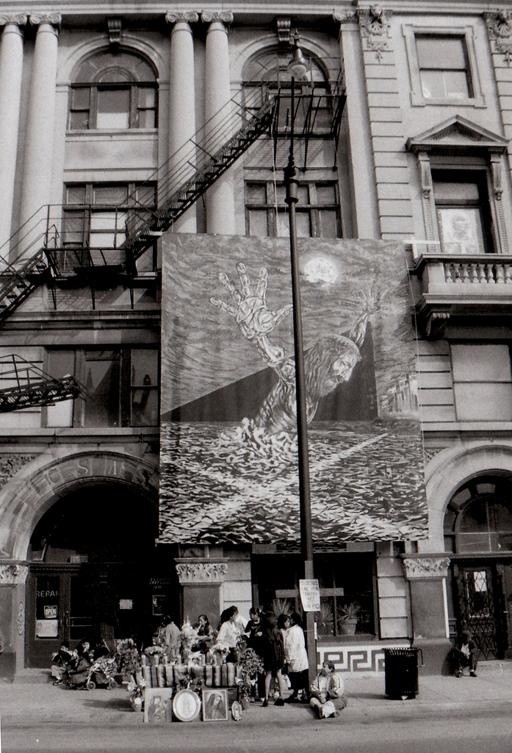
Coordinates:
[382,647,422,700]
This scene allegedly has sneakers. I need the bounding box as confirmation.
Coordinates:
[314,704,323,719]
[250,695,309,707]
[455,671,478,678]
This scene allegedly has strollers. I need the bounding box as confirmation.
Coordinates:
[64,646,118,690]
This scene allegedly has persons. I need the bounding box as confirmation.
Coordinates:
[308,660,347,720]
[453,630,479,677]
[51,637,110,686]
[211,261,394,440]
[161,605,308,707]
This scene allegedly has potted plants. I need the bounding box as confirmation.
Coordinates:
[338,601,362,635]
